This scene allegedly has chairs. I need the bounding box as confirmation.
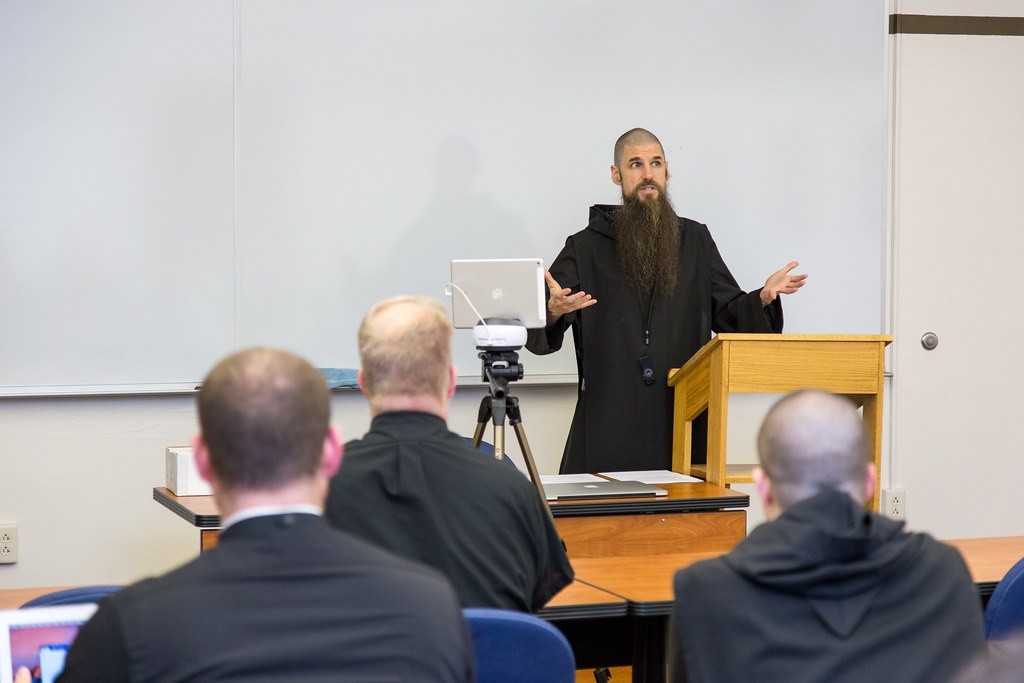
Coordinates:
[18,587,123,606]
[983,556,1024,642]
[462,604,576,683]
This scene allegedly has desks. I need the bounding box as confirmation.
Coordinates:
[0,579,626,633]
[154,475,751,558]
[570,535,1024,683]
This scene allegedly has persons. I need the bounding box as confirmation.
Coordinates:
[321,297,574,616]
[15,346,473,683]
[666,388,989,683]
[525,127,808,483]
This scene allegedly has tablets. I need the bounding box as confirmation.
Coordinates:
[450,258,547,330]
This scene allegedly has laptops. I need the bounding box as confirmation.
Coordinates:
[543,480,668,501]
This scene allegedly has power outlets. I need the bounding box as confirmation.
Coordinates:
[0,525,19,564]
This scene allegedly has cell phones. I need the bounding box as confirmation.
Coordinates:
[640,356,655,385]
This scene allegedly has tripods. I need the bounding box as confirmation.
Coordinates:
[471,319,558,538]
